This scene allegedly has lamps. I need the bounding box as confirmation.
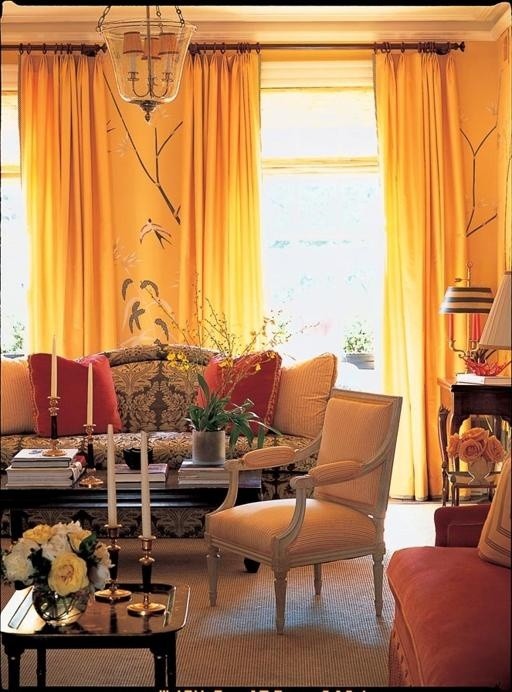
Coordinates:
[94,4,194,125]
[476,267,512,353]
[441,261,494,373]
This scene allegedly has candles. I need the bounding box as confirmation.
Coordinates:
[103,422,120,529]
[139,430,152,538]
[84,357,94,428]
[49,345,59,399]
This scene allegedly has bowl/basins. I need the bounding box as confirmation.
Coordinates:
[123,447,154,469]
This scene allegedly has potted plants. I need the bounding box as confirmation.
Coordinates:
[184,375,277,468]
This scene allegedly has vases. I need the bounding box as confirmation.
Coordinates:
[31,585,90,627]
[464,457,492,486]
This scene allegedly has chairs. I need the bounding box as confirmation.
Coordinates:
[200,385,404,637]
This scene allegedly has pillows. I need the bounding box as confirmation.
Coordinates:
[270,352,341,440]
[476,447,510,569]
[192,350,279,436]
[26,350,122,441]
[0,357,44,439]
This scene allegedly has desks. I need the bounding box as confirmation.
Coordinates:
[2,458,268,581]
[434,371,510,511]
[442,471,498,507]
[2,578,194,687]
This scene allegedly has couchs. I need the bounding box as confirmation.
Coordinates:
[0,341,337,576]
[382,494,512,690]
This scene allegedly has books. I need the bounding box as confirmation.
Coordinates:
[5,449,89,487]
[456,373,511,387]
[115,462,168,484]
[176,460,264,488]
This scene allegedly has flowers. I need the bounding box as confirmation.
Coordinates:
[146,286,323,398]
[2,522,113,608]
[444,426,508,463]
